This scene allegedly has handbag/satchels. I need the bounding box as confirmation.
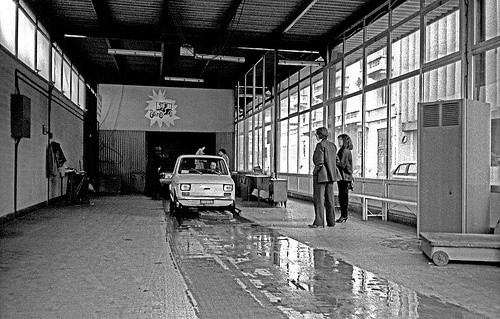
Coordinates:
[348,177,354,191]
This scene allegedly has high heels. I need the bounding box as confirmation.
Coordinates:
[335,217,348,223]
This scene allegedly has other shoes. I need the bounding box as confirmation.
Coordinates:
[327,224,335,227]
[308,224,324,228]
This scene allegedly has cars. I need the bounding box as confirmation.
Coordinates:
[159,154,238,221]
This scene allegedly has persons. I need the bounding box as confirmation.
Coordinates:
[195,146,207,169]
[308,127,337,228]
[204,161,222,175]
[335,134,353,223]
[218,149,229,171]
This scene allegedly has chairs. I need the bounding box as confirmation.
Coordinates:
[182,163,196,170]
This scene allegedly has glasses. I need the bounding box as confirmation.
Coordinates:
[315,133,318,135]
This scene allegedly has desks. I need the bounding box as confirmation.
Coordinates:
[271,179,288,208]
[245,175,272,206]
